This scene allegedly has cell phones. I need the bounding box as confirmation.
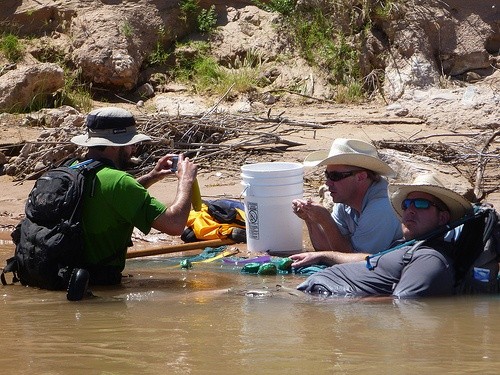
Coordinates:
[171,156,178,171]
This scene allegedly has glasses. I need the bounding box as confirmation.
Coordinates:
[401,198,444,212]
[324,169,356,182]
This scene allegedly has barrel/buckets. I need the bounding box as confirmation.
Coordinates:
[239,160,306,254]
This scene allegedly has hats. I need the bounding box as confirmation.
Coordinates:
[304,138,396,179]
[387,175,471,221]
[70,107,152,147]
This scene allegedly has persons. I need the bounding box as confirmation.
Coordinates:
[67,109,200,288]
[292,138,405,254]
[297,175,476,301]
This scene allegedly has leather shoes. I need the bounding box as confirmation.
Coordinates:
[67,268,100,301]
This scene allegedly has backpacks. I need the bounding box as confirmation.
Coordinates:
[449,208,500,293]
[0,157,117,290]
